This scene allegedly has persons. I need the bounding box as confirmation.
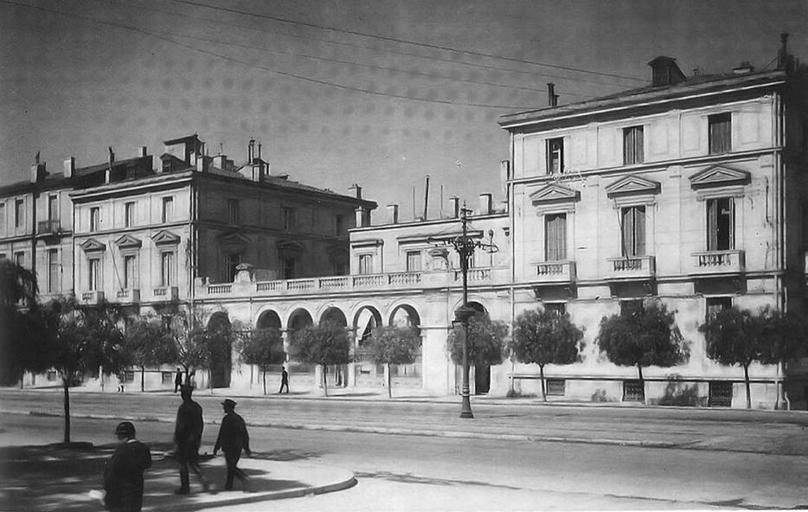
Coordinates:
[174,385,211,495]
[174,368,182,393]
[279,367,289,393]
[103,422,151,512]
[214,399,251,491]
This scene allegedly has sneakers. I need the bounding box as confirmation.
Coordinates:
[243,477,250,492]
[174,487,189,494]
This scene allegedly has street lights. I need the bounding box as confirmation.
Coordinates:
[424,200,497,419]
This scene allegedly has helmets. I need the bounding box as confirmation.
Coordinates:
[115,422,136,435]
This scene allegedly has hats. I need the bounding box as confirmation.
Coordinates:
[178,384,194,390]
[221,399,237,406]
